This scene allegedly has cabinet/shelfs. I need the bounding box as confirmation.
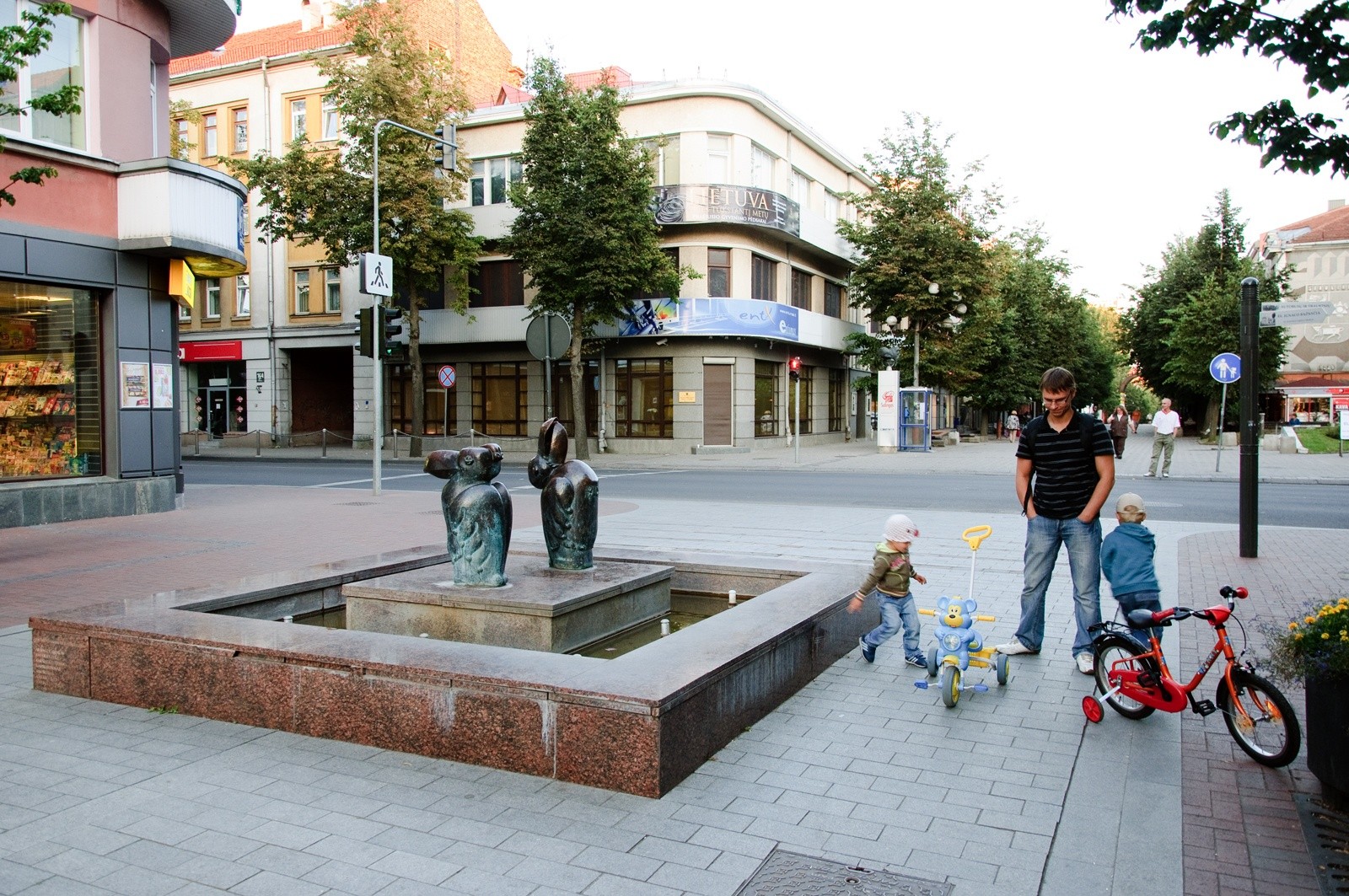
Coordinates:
[0,384,75,423]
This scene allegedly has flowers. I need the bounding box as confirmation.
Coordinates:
[1248,589,1349,700]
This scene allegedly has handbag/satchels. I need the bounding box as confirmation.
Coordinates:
[1006,425,1010,430]
[1108,429,1113,439]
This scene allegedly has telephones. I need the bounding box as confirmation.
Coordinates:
[904,407,910,418]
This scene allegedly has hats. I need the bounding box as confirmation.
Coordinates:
[1116,492,1146,514]
[882,514,919,542]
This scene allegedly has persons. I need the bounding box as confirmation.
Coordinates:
[761,410,772,434]
[1006,410,1020,443]
[1146,413,1152,424]
[1107,405,1134,459]
[848,514,927,669]
[1289,413,1299,425]
[1143,398,1181,477]
[996,367,1117,674]
[1023,411,1032,425]
[1101,492,1164,647]
[1324,412,1338,422]
[1092,405,1099,417]
[1132,408,1140,434]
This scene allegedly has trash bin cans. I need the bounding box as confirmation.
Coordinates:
[1259,412,1265,439]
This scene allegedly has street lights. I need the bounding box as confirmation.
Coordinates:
[885,282,967,445]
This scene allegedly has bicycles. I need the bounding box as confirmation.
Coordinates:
[1082,585,1302,768]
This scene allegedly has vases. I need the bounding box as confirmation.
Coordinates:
[1301,647,1349,816]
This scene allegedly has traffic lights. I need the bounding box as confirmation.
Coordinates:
[354,307,373,359]
[378,305,404,358]
[433,125,457,172]
[789,358,800,380]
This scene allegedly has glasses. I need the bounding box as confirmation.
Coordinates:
[1043,387,1072,404]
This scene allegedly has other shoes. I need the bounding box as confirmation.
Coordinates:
[1163,473,1169,477]
[1144,472,1154,477]
[1115,456,1122,459]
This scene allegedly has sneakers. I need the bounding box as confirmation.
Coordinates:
[996,633,1039,656]
[906,653,927,668]
[1075,652,1095,675]
[858,634,876,663]
